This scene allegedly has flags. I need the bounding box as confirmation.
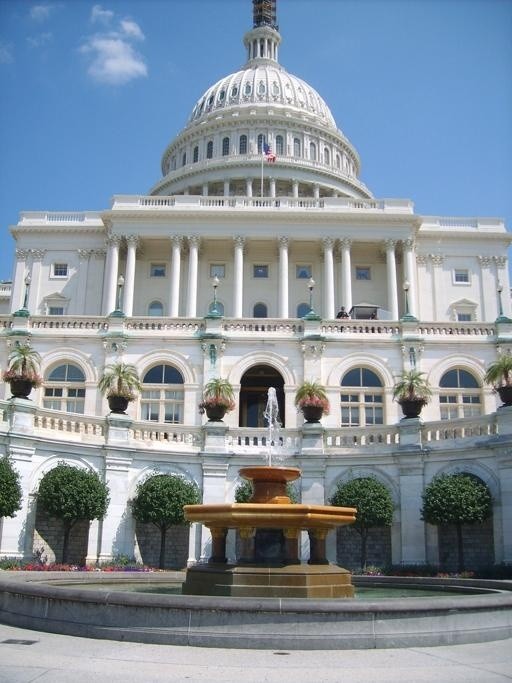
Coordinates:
[262,132,277,161]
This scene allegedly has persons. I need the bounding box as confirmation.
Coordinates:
[369,311,379,332]
[337,306,350,333]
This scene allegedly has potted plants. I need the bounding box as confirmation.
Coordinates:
[391,346,512,418]
[198,376,331,425]
[3,343,147,413]
